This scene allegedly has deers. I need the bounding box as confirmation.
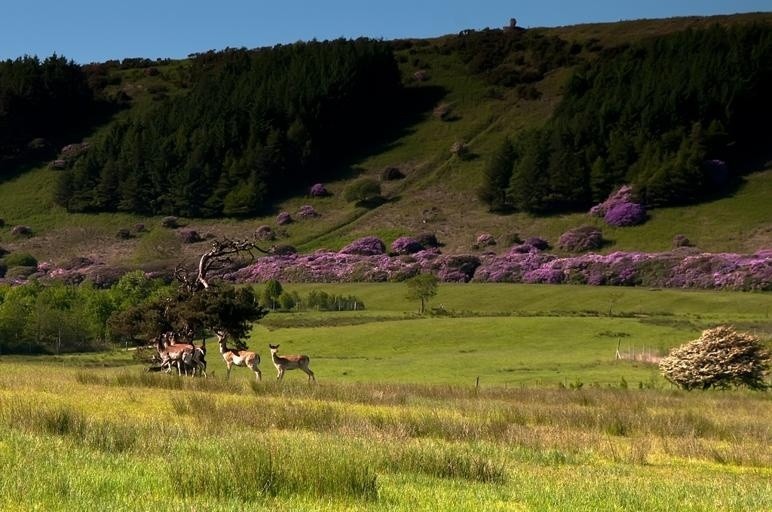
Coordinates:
[269,343,316,384]
[155,333,208,380]
[214,328,261,384]
